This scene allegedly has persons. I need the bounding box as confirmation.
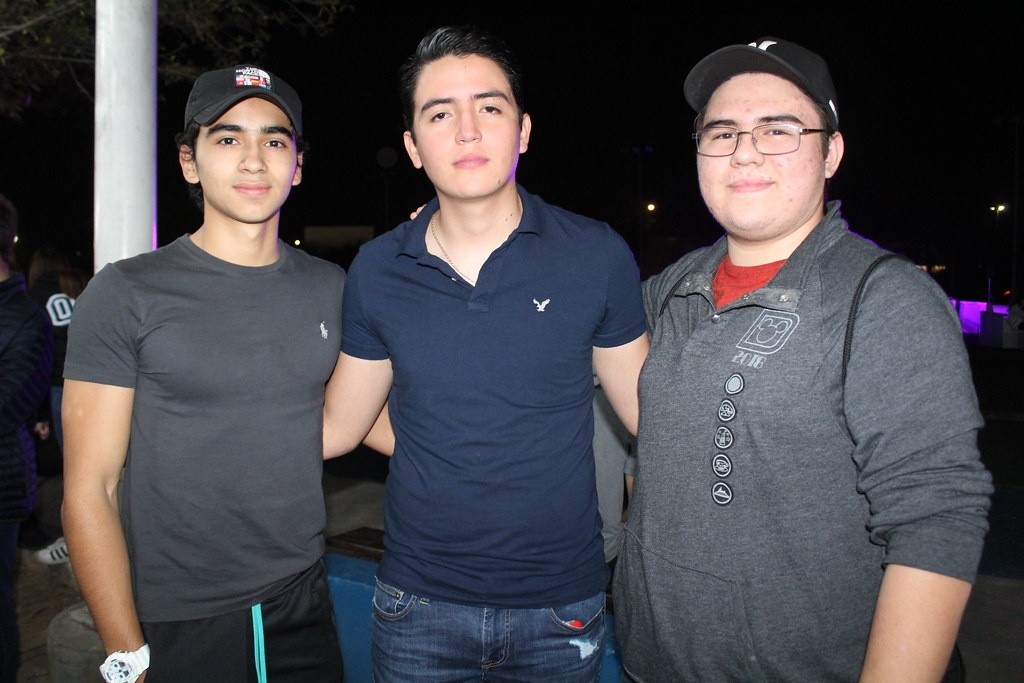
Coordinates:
[60,64,395,683]
[16,245,87,565]
[591,34,995,682]
[321,33,651,681]
[0,197,53,683]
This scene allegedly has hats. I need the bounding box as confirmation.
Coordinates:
[684,39,837,138]
[185,64,302,139]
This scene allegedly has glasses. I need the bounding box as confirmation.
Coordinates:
[691,122,833,159]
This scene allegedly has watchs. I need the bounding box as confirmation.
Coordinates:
[99,643,150,683]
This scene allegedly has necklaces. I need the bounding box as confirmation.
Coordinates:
[431,212,476,287]
